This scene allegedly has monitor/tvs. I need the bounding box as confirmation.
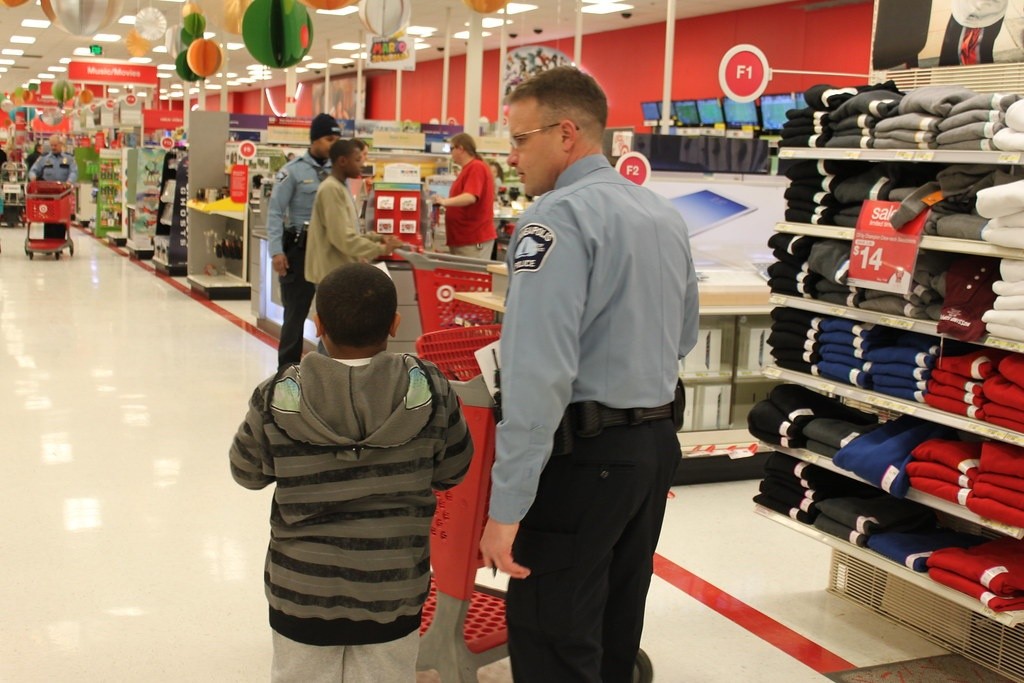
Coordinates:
[639,92,810,136]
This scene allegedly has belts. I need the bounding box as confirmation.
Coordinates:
[570,405,671,431]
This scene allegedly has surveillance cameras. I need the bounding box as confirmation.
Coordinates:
[342,64,354,68]
[437,48,444,51]
[509,33,516,38]
[621,13,630,18]
[315,71,320,73]
[534,29,542,34]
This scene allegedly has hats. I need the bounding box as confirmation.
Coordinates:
[310,113,341,141]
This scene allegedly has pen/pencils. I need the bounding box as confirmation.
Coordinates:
[492,565,497,577]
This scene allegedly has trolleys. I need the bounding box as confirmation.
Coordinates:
[422,322,654,676]
[21,179,78,259]
[391,245,511,336]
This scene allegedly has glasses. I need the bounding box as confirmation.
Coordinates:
[450,146,455,151]
[511,123,580,149]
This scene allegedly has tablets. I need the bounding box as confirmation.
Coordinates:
[669,189,759,237]
[750,260,776,282]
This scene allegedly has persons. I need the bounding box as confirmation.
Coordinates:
[488,162,504,260]
[267,113,343,372]
[305,138,399,358]
[432,134,498,260]
[0,144,8,174]
[29,135,78,255]
[27,143,45,181]
[229,262,475,683]
[479,66,699,683]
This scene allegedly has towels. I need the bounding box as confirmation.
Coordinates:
[746,76,1024,613]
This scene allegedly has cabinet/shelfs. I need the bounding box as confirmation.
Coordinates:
[186,187,250,299]
[747,1,1024,683]
